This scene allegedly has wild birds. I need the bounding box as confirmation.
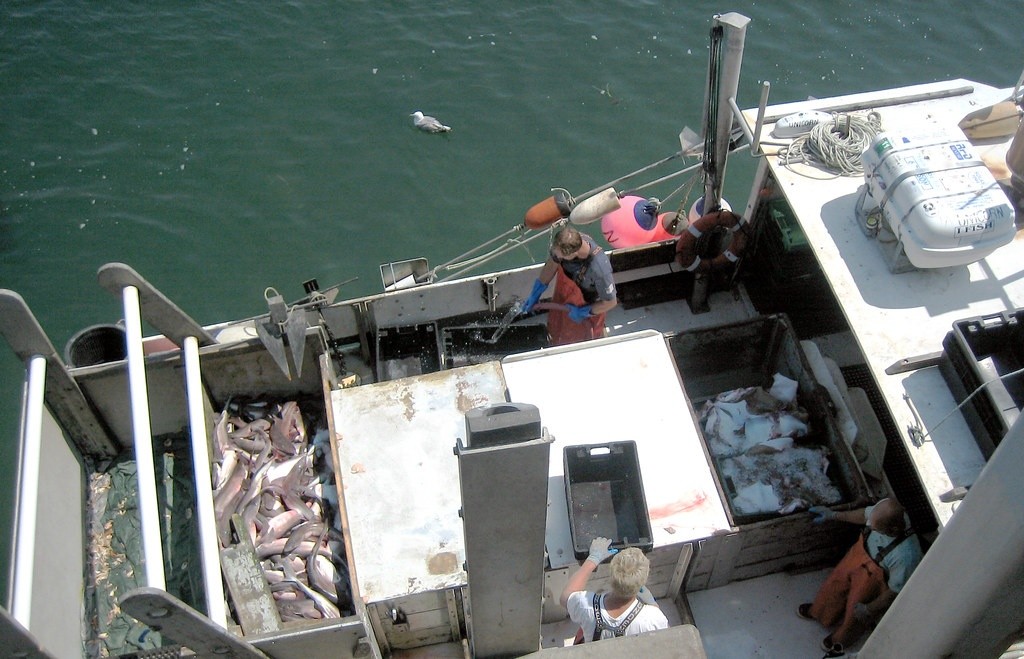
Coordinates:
[410,111,452,133]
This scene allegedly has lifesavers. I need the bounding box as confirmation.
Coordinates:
[676,212,747,274]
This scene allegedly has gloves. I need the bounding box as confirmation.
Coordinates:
[522,278,548,312]
[566,304,596,325]
[852,603,872,622]
[810,506,837,523]
[637,586,659,607]
[587,537,618,567]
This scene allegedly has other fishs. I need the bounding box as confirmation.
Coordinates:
[214,385,351,622]
[699,373,842,515]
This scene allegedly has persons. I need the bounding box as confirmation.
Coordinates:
[521,226,617,347]
[559,537,668,646]
[797,498,922,652]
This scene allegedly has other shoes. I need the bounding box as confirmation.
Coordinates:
[821,633,834,652]
[798,603,815,621]
